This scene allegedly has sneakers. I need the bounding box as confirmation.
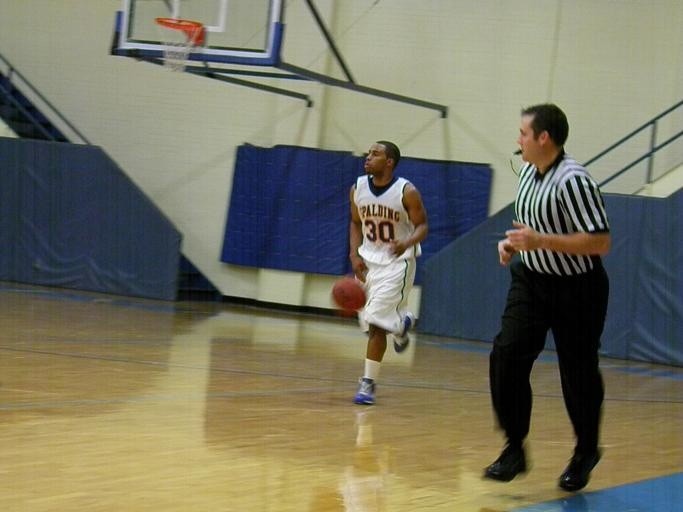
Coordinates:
[353,376,376,405]
[393,312,415,352]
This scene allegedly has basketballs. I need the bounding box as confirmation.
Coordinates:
[334,278,366,310]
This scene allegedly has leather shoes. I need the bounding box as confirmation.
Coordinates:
[485,443,526,481]
[558,439,600,491]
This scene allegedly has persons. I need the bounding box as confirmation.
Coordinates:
[485,104,612,492]
[348,140,429,405]
